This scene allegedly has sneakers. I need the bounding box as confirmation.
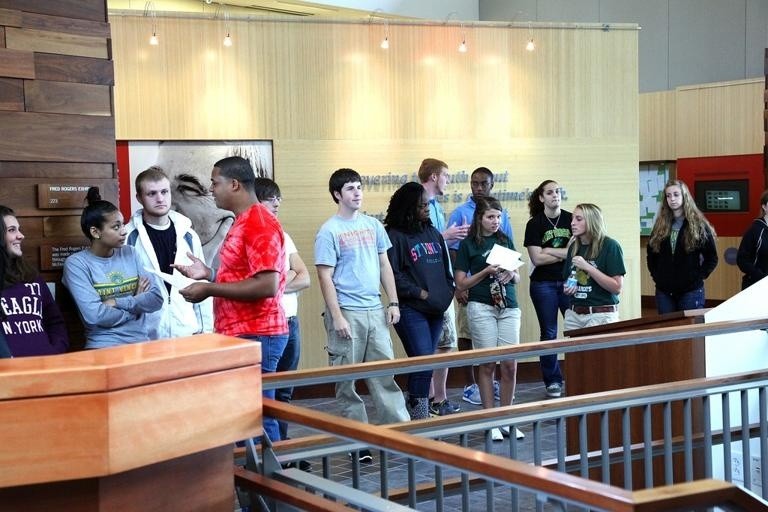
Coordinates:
[547,384,562,396]
[427,400,464,418]
[494,381,515,401]
[282,461,312,473]
[492,426,525,441]
[350,450,374,462]
[463,383,482,405]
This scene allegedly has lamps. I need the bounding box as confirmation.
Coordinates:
[140,0,536,54]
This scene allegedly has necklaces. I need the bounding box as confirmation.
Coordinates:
[546,215,560,230]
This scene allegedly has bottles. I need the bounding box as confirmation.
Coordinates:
[567,270,578,289]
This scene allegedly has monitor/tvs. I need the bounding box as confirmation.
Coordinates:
[693,178,750,213]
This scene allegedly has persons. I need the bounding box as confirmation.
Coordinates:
[313,158,525,460]
[523,180,577,397]
[736,190,768,290]
[561,203,627,330]
[0,157,312,473]
[647,180,719,314]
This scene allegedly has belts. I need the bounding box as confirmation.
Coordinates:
[568,304,615,314]
